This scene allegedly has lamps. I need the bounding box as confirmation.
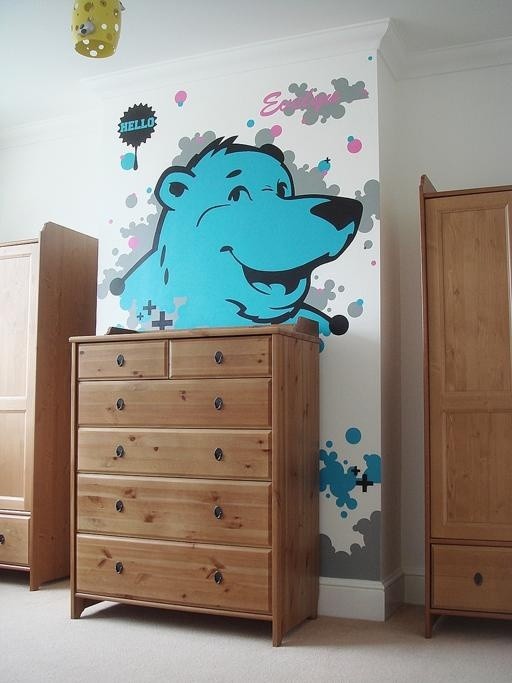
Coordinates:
[72,0,125,59]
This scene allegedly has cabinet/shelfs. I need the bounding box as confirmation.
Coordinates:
[0,221,98,591]
[419,174,512,639]
[69,317,321,647]
